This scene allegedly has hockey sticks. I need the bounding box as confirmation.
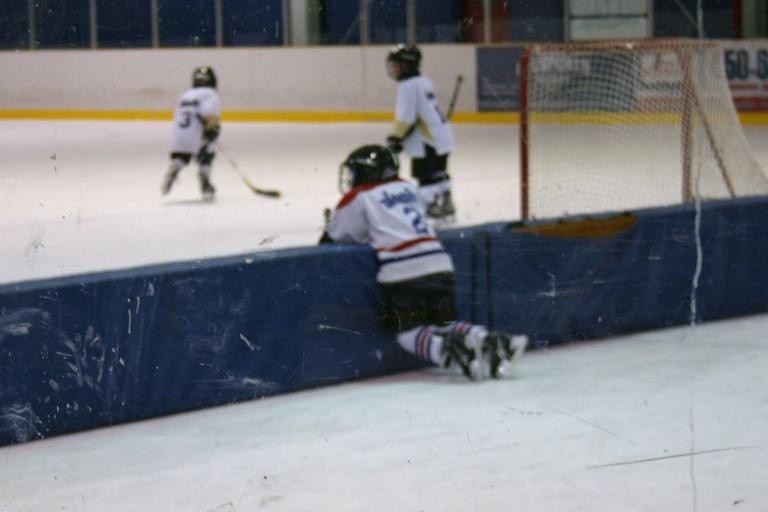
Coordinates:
[214,140,282,197]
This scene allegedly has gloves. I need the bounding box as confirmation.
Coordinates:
[388,137,403,153]
[203,125,219,141]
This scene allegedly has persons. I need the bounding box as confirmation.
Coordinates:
[160,65,222,196]
[318,144,530,381]
[385,41,456,218]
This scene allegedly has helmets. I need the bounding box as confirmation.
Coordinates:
[193,67,216,88]
[388,43,420,80]
[339,144,398,196]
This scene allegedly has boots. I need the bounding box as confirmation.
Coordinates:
[201,180,214,192]
[444,321,475,380]
[482,333,517,376]
[426,191,454,217]
